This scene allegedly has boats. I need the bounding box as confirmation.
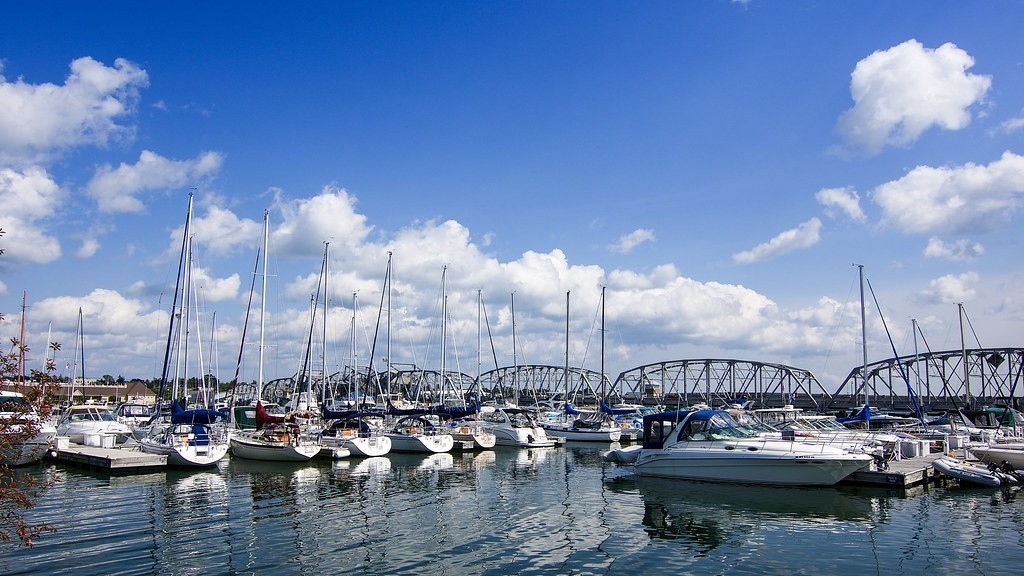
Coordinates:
[602,410,919,487]
[931,454,1024,494]
[993,433,1024,444]
[110,401,153,428]
[968,444,1024,473]
[283,391,319,413]
[989,440,1024,450]
[330,395,375,408]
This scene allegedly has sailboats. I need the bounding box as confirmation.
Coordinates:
[535,290,621,443]
[361,249,454,453]
[445,287,557,448]
[817,264,1023,465]
[140,186,235,467]
[228,208,322,461]
[289,240,392,456]
[0,287,57,471]
[573,286,676,440]
[56,306,132,444]
[412,263,497,450]
